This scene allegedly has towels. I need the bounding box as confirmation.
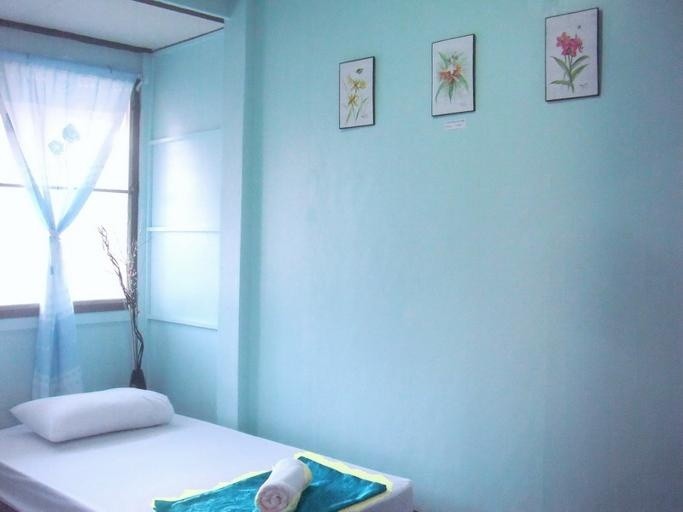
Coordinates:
[252,454,315,510]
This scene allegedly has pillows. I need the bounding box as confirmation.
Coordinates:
[6,380,177,446]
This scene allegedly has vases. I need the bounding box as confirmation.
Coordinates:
[125,368,148,389]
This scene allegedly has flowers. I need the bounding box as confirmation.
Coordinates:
[548,22,592,97]
[433,46,469,106]
[341,65,371,124]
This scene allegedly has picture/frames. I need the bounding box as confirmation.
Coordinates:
[543,5,602,105]
[335,52,376,132]
[429,31,478,119]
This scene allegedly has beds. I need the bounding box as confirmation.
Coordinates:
[1,415,417,511]
